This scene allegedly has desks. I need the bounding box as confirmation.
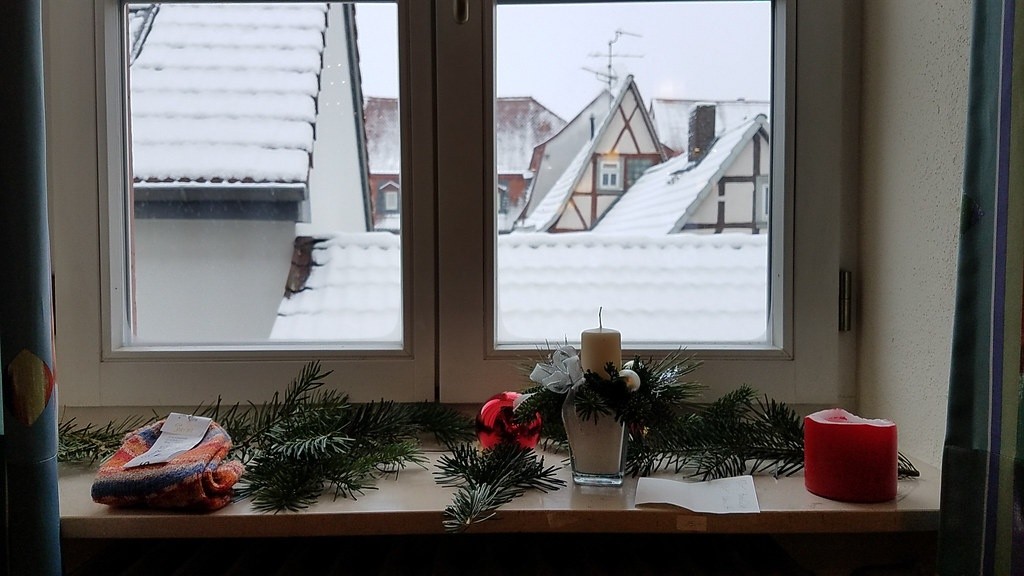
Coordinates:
[54,426,935,539]
